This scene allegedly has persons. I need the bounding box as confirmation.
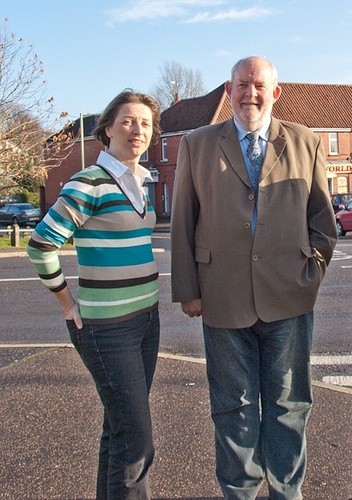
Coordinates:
[26,87,161,500]
[171,56,337,500]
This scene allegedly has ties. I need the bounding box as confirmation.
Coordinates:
[246,133,263,207]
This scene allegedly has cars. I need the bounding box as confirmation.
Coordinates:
[0,203,41,227]
[330,193,352,214]
[335,200,352,236]
